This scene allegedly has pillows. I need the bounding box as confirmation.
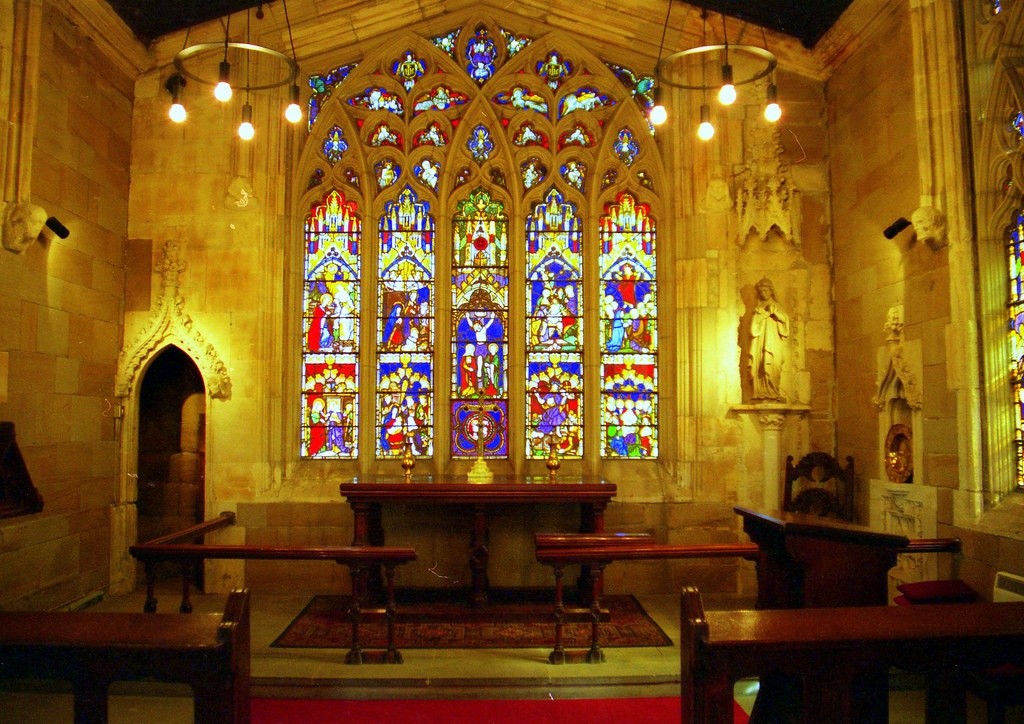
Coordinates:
[892,594,982,608]
[895,578,979,603]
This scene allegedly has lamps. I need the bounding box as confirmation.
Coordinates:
[883,216,912,239]
[169,0,302,140]
[45,216,70,240]
[652,0,782,143]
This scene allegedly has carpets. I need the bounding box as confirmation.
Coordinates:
[269,594,675,648]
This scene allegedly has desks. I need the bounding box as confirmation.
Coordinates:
[340,474,618,620]
[678,585,1024,724]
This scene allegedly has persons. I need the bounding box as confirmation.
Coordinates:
[746,278,790,404]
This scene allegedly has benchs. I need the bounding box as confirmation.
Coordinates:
[0,586,252,724]
